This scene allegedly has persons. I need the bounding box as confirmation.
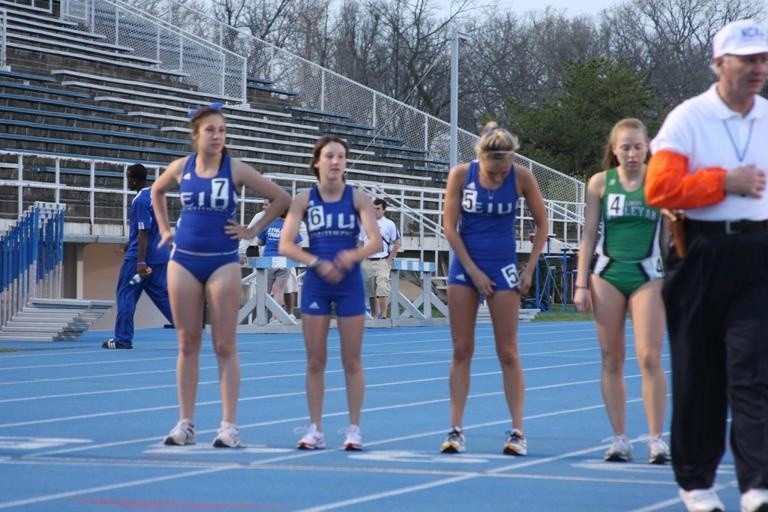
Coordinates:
[441,121,549,456]
[151,102,292,448]
[358,198,400,320]
[101,164,173,349]
[277,136,383,450]
[645,18,768,512]
[238,196,310,323]
[573,117,673,465]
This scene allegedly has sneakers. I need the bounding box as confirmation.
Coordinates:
[647,438,672,464]
[679,487,725,512]
[297,424,326,449]
[101,338,133,349]
[502,429,528,456]
[211,426,240,448]
[343,424,362,450]
[163,420,196,446]
[740,488,768,512]
[439,424,466,453]
[602,434,633,462]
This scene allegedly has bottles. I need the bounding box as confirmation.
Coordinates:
[129,266,152,286]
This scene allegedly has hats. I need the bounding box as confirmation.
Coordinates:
[712,19,768,60]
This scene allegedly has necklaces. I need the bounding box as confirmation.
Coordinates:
[720,118,755,197]
[487,184,497,199]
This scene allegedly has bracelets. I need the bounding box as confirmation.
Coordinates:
[575,286,588,289]
[138,261,146,265]
[307,256,322,271]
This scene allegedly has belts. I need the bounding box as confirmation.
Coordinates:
[683,219,768,234]
[366,256,389,261]
